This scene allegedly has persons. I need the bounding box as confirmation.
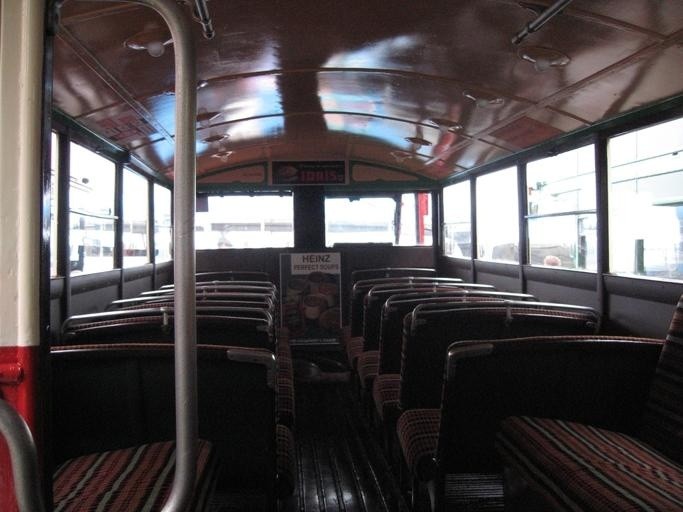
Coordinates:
[295,273,331,336]
[542,255,561,267]
